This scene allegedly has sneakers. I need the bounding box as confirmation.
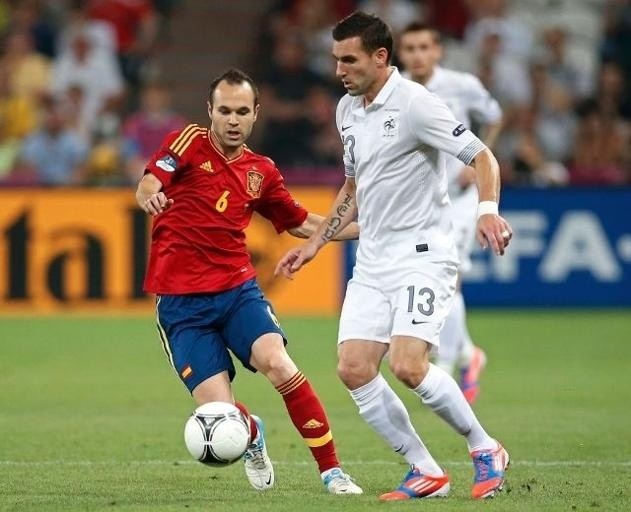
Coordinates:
[242,414,274,490]
[379,463,450,501]
[469,440,510,499]
[320,467,363,495]
[459,347,487,404]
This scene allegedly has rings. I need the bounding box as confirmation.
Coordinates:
[502,230,509,239]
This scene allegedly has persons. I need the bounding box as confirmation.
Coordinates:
[363,1,630,184]
[394,18,506,406]
[134,63,366,497]
[0,0,188,190]
[253,0,343,170]
[273,7,512,502]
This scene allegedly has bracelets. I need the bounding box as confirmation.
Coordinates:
[476,201,500,221]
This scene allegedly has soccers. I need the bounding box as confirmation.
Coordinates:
[184,401,249,467]
